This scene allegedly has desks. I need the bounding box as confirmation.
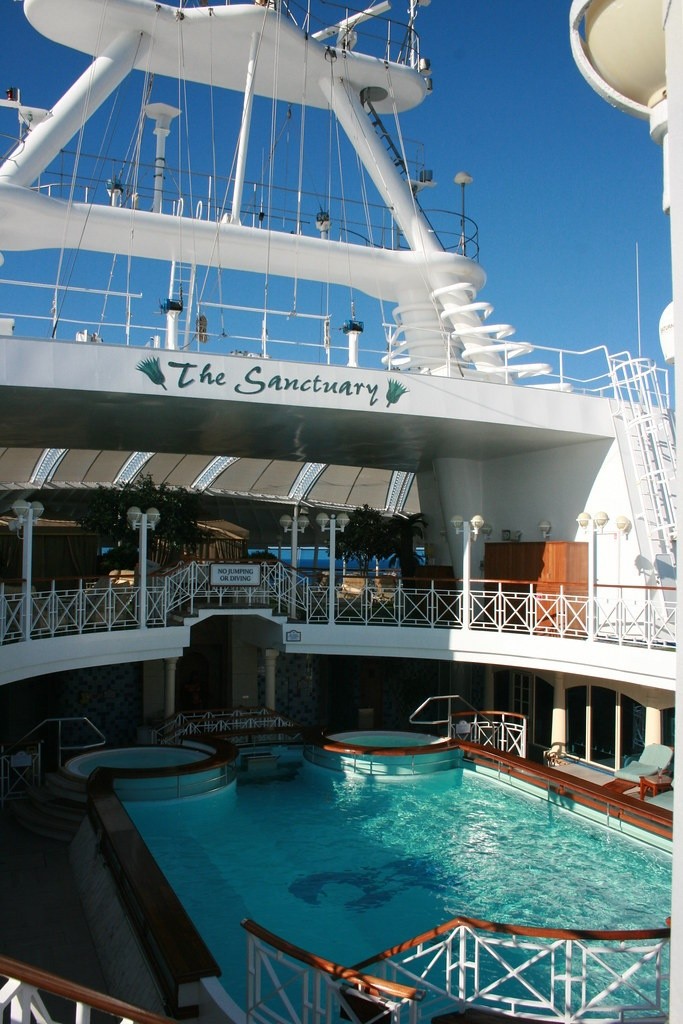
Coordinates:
[640,775,672,800]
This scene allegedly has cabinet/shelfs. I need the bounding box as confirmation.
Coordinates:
[485,541,588,636]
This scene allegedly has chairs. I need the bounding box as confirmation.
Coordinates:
[645,779,673,812]
[614,744,673,783]
